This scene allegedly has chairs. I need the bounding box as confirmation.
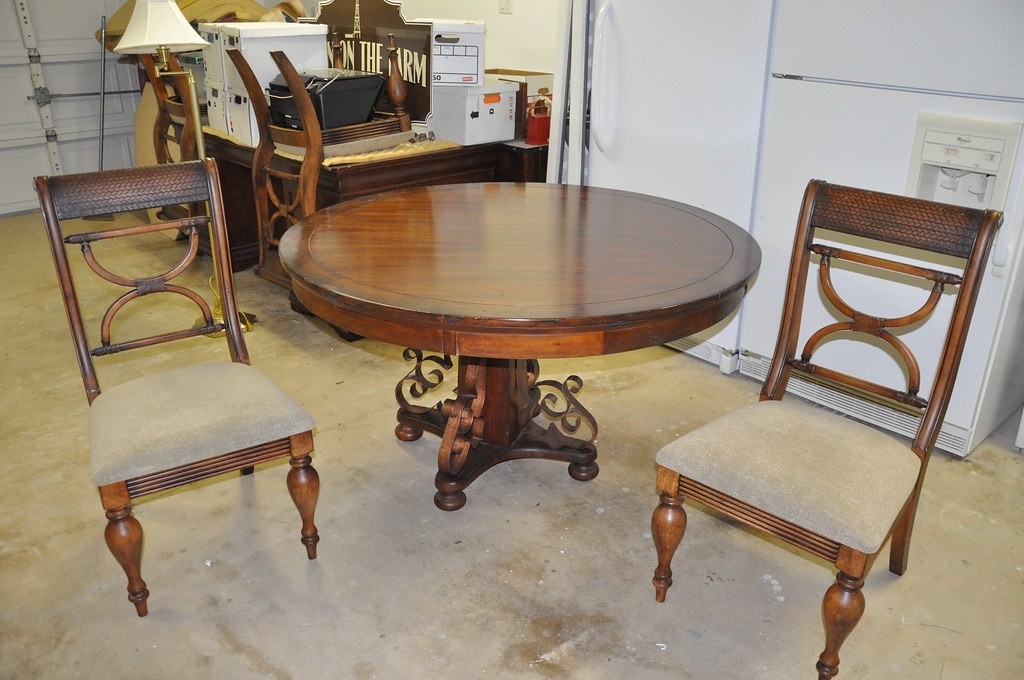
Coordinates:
[31,157,320,618]
[653,179,1004,680]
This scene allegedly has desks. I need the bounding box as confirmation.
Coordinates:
[495,139,548,181]
[278,182,761,512]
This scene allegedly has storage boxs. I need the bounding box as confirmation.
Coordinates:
[410,17,486,86]
[178,22,330,148]
[410,80,520,146]
[484,68,554,138]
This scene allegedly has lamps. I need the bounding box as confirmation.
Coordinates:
[113,0,258,338]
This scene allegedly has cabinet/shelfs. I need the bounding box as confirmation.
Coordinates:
[169,142,502,291]
